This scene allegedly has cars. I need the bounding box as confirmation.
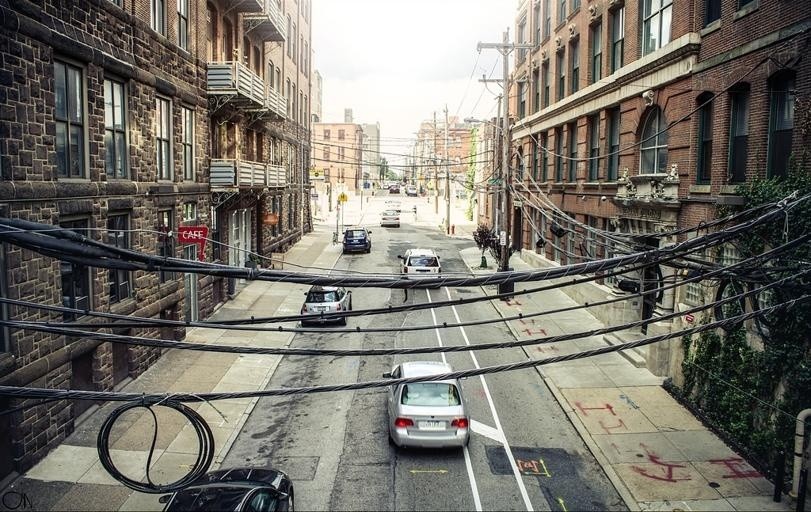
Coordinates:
[379,211,401,227]
[343,227,373,254]
[385,200,402,212]
[159,465,295,511]
[301,285,353,328]
[382,180,418,197]
[383,360,471,449]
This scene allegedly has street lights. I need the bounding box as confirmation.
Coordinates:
[464,119,510,276]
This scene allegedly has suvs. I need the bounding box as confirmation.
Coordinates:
[398,248,443,289]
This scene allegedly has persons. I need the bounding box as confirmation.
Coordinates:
[412,205,418,216]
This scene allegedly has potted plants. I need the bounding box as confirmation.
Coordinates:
[472,223,494,267]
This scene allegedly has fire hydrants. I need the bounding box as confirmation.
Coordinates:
[449,224,456,236]
